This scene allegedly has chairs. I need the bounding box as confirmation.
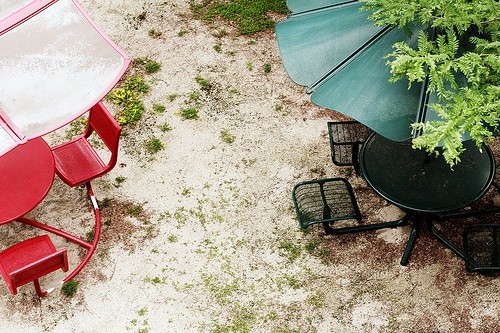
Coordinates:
[49,101,122,188]
[0,235,69,298]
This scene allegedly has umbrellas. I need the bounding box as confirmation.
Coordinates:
[1,1,131,160]
[273,1,500,146]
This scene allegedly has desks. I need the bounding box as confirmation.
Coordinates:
[359,130,496,214]
[0,136,55,226]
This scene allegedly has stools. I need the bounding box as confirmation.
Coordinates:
[292,178,363,228]
[326,120,374,167]
